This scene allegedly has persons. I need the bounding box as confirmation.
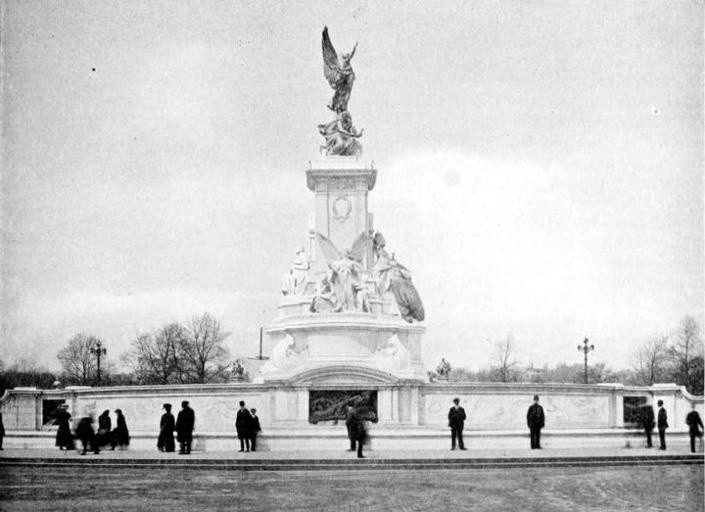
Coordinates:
[250,408,263,450]
[355,416,366,458]
[685,402,705,452]
[329,248,363,313]
[345,406,360,452]
[526,394,545,449]
[322,26,359,114]
[55,404,129,456]
[656,399,669,449]
[374,232,398,279]
[447,397,468,450]
[175,400,195,454]
[156,402,176,452]
[383,257,417,324]
[235,400,255,451]
[641,398,656,447]
[0,412,5,451]
[318,112,363,157]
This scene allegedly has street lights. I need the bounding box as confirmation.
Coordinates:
[90,340,109,388]
[577,337,596,385]
